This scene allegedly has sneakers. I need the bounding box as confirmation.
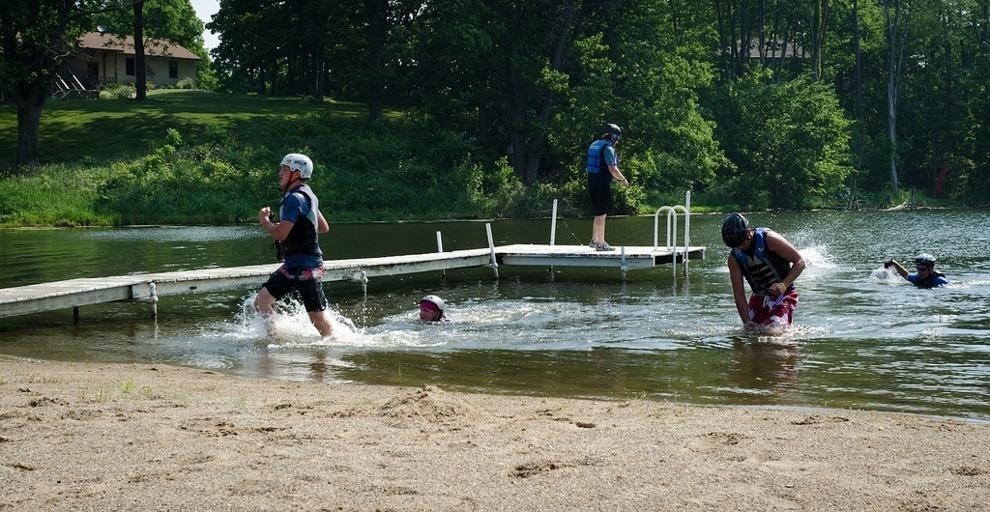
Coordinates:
[588,240,615,251]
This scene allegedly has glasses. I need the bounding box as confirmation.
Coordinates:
[917,268,927,271]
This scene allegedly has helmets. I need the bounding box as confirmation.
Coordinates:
[916,253,936,266]
[280,153,314,178]
[605,123,622,135]
[420,295,445,312]
[722,212,750,247]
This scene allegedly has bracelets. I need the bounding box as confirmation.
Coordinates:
[620,177,627,183]
[779,281,788,292]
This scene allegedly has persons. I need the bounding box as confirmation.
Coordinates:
[882,252,949,290]
[585,122,630,252]
[720,210,808,333]
[251,152,343,345]
[418,295,447,326]
